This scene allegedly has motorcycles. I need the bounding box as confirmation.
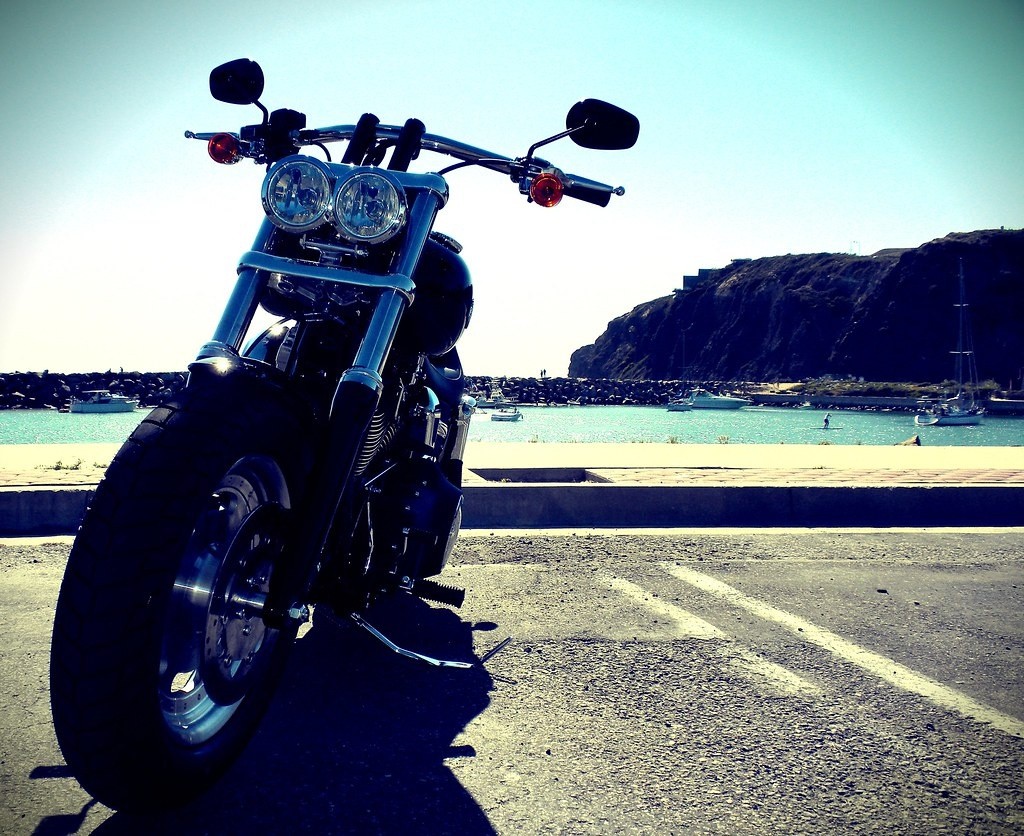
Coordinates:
[50,56,640,817]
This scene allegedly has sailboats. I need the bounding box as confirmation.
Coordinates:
[913,256,986,426]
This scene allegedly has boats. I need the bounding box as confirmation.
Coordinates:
[667,397,694,411]
[667,389,754,409]
[476,379,519,409]
[70,390,141,413]
[491,403,522,422]
